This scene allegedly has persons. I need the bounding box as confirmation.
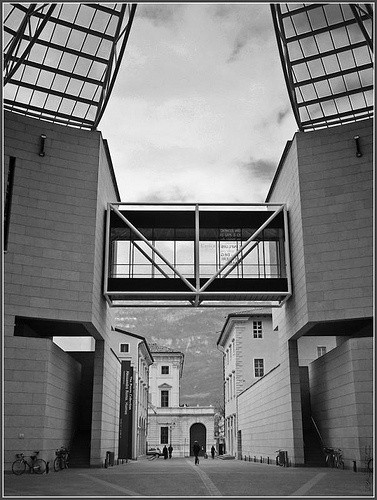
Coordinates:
[163,445,168,459]
[193,441,201,465]
[168,445,173,459]
[211,445,217,459]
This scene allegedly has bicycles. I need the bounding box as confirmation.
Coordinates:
[324,447,345,470]
[276,449,288,466]
[54,447,72,472]
[12,450,47,475]
[368,459,373,472]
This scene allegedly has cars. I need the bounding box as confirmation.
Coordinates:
[147,447,161,456]
